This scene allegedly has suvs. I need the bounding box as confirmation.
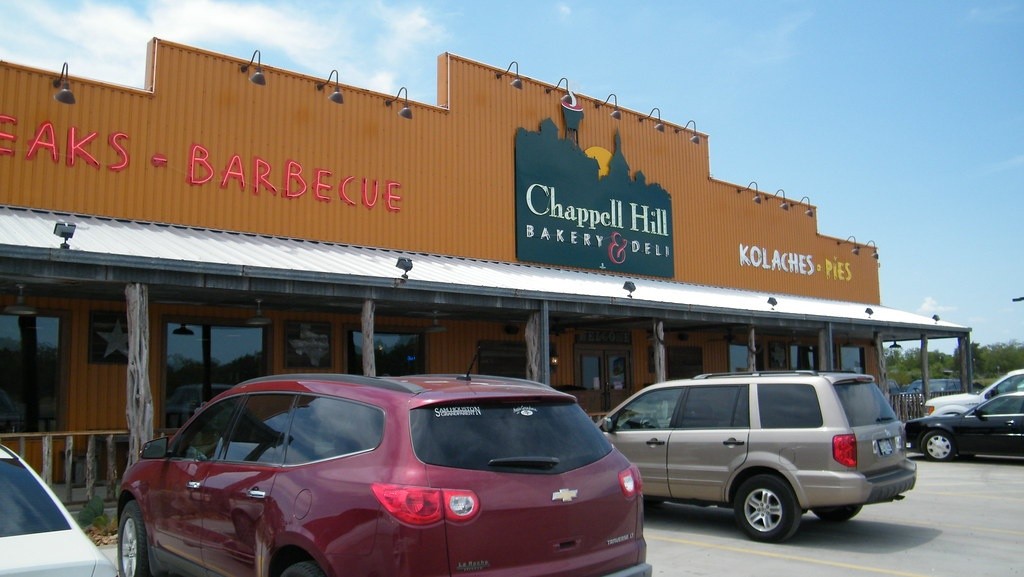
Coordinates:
[161,383,229,418]
[920,368,1024,417]
[115,372,652,577]
[593,369,916,543]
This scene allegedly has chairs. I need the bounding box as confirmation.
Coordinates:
[679,400,718,428]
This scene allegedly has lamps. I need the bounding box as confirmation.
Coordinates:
[842,337,857,347]
[1,283,40,315]
[837,235,859,256]
[866,307,873,319]
[863,240,879,260]
[497,61,522,89]
[767,296,777,310]
[738,181,761,204]
[790,196,814,218]
[889,341,901,348]
[243,298,273,325]
[52,62,76,105]
[675,120,700,144]
[503,324,520,336]
[395,255,415,280]
[171,324,194,336]
[241,49,266,86]
[678,333,690,341]
[595,94,621,120]
[623,281,636,297]
[549,320,565,336]
[546,78,572,105]
[723,329,737,344]
[638,107,664,132]
[422,319,448,334]
[788,337,801,346]
[53,222,77,250]
[765,189,788,211]
[549,351,561,370]
[386,87,412,120]
[317,70,344,104]
[932,314,940,324]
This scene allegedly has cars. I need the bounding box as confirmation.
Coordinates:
[904,378,961,396]
[0,443,118,577]
[887,379,903,395]
[903,391,1024,461]
